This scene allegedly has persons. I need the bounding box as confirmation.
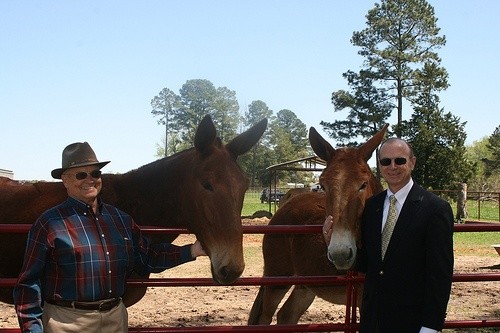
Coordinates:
[13,142,208,333]
[322,137,455,333]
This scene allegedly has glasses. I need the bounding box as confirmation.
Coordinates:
[66,169,102,180]
[379,157,409,166]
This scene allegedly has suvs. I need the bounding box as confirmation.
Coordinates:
[261,189,284,204]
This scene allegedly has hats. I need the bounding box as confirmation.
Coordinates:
[51,141,110,179]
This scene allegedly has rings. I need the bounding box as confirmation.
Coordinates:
[324,230,328,234]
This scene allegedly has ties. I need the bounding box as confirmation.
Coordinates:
[381,195,398,261]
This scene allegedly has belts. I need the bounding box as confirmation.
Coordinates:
[45,297,122,312]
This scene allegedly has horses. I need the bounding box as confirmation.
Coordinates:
[247,122,390,326]
[0,114,268,309]
